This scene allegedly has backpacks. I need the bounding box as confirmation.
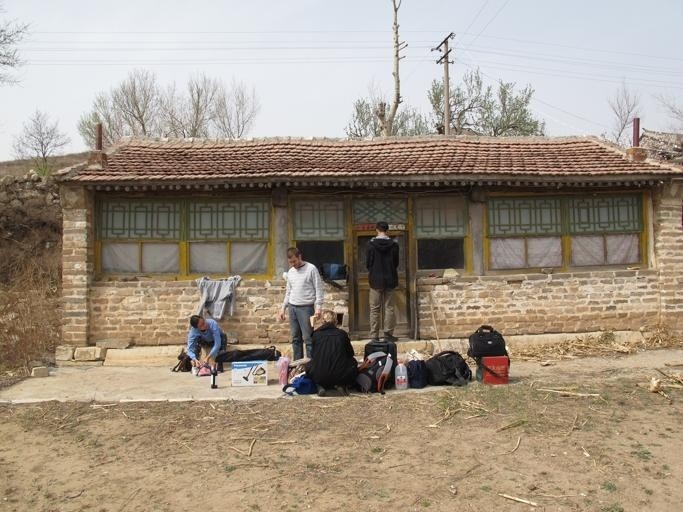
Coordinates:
[354,351,394,394]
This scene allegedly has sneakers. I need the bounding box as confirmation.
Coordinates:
[317,386,326,396]
[383,332,398,343]
[210,368,217,376]
[336,384,350,396]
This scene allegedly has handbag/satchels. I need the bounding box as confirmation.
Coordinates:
[318,263,350,288]
[426,351,472,386]
[172,348,192,372]
[283,374,318,396]
[277,347,291,385]
[407,360,429,388]
[467,325,510,377]
[364,340,397,367]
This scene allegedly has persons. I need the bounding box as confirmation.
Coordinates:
[364,221,400,342]
[187,315,228,374]
[277,247,324,360]
[307,311,357,397]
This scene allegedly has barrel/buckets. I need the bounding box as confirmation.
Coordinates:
[395,361,408,389]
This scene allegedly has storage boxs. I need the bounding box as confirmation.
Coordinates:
[230,360,268,386]
